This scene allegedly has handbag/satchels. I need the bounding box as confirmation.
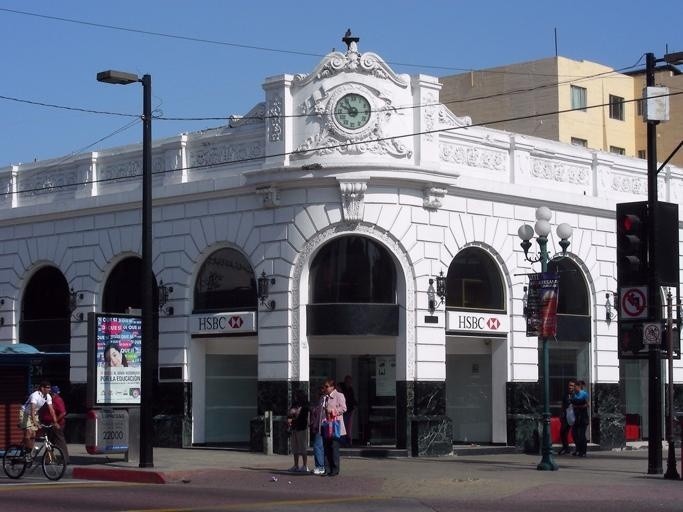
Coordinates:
[319,419,341,441]
[284,415,298,434]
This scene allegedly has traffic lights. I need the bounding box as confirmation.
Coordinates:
[615,200,649,287]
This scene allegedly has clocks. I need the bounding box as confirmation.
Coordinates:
[334,93,371,130]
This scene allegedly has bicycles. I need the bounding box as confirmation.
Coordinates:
[2,423,66,481]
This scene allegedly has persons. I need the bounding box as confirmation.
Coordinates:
[18,382,60,463]
[38,385,72,467]
[558,376,576,456]
[569,381,590,457]
[287,388,309,473]
[309,387,326,476]
[103,343,128,366]
[317,379,349,478]
[336,375,361,447]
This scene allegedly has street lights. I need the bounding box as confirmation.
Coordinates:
[517,205,573,470]
[96,68,154,469]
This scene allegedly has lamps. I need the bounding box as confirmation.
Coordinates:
[67,287,83,321]
[606,295,618,320]
[258,268,275,311]
[157,280,173,317]
[429,271,448,309]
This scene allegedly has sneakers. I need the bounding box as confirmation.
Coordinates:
[558,448,586,457]
[25,452,31,463]
[287,465,339,477]
